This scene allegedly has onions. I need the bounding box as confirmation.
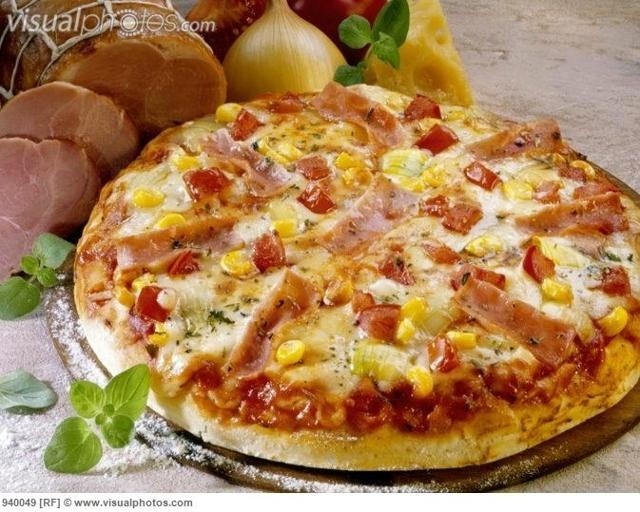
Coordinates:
[222,0,350,103]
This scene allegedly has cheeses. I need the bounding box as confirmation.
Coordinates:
[363,0,473,107]
